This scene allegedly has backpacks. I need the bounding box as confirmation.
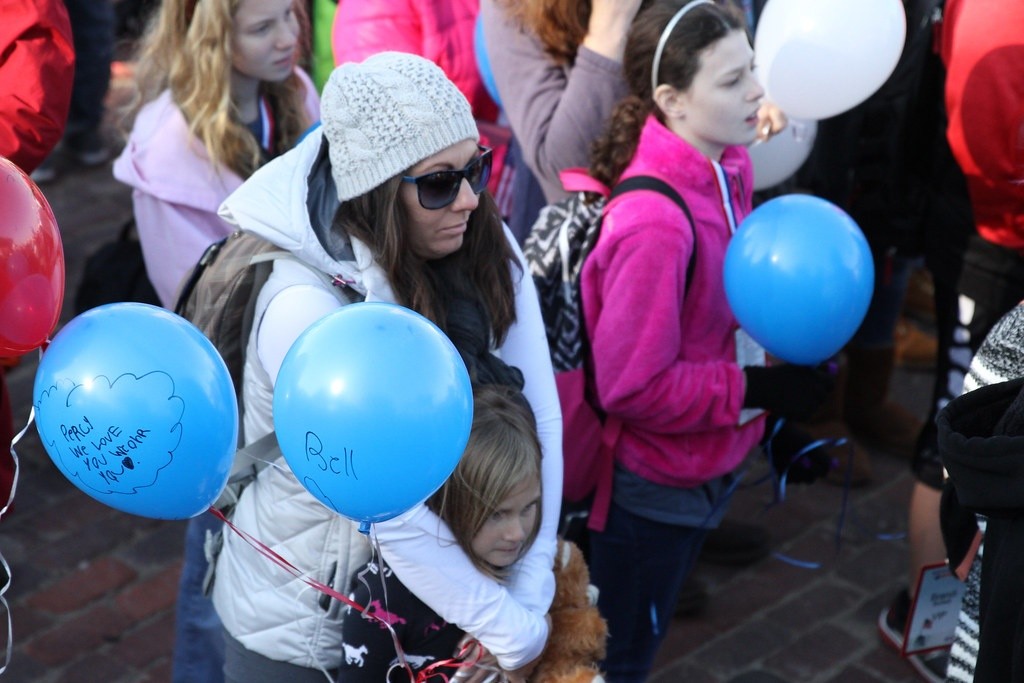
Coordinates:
[173,227,365,518]
[520,164,698,372]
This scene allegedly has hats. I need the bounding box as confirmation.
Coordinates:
[318,50,479,202]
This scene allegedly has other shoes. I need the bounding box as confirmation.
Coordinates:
[698,520,770,564]
[881,590,951,682]
[800,418,875,485]
[32,156,60,182]
[855,395,927,456]
[73,135,123,166]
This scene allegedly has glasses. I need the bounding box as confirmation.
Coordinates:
[401,145,492,210]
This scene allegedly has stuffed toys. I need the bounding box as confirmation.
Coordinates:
[530,535,611,683]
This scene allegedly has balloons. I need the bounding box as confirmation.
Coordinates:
[723,195,875,367]
[754,1,906,119]
[0,155,64,372]
[272,302,474,534]
[34,303,238,518]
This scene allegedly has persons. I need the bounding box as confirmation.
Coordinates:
[0,0,754,683]
[875,0,1024,683]
[474,1,818,683]
